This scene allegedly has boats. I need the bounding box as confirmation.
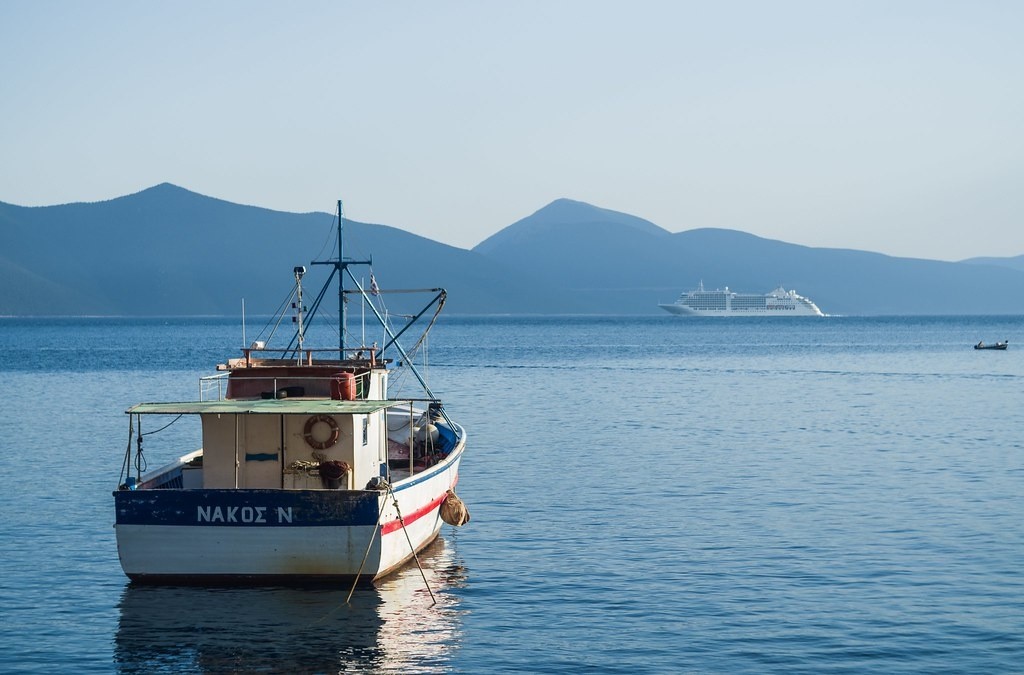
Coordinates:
[658,278,824,317]
[974,340,1008,349]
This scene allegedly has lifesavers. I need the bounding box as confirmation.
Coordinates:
[303,414,340,450]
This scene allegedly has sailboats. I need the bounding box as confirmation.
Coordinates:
[111,200,468,591]
